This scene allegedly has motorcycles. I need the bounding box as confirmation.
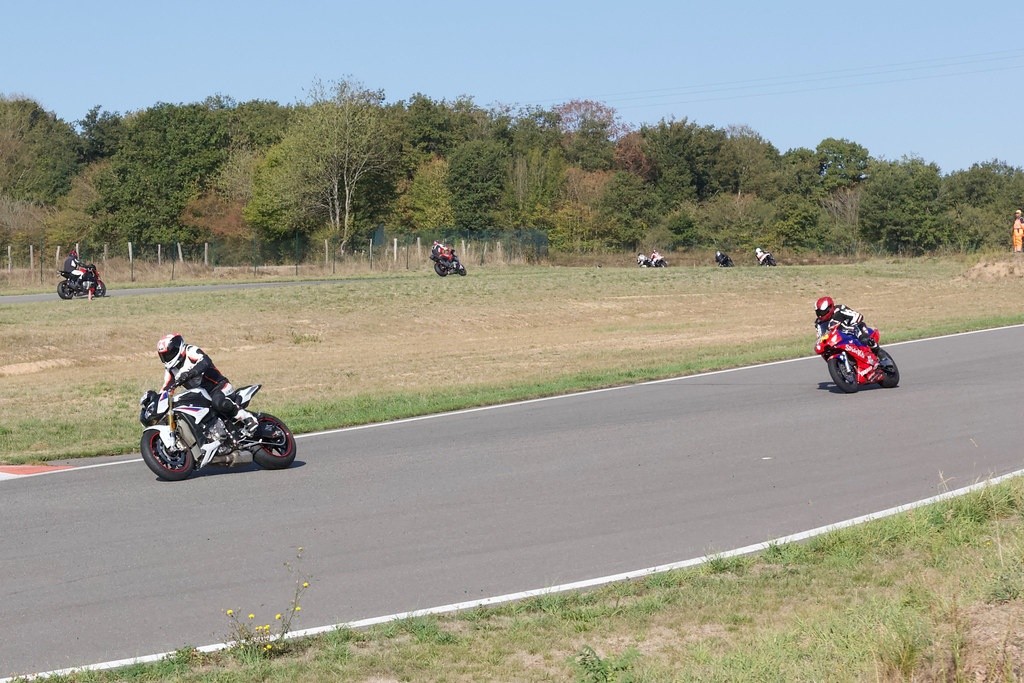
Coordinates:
[56,261,106,300]
[814,322,900,394]
[717,254,734,267]
[138,375,297,482]
[637,256,669,267]
[429,249,467,277]
[758,252,777,267]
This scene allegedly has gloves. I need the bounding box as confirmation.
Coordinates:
[179,369,198,384]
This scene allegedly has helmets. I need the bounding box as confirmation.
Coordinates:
[433,240,440,245]
[756,248,762,254]
[157,332,186,370]
[653,250,656,253]
[814,296,834,322]
[638,253,642,256]
[69,249,77,260]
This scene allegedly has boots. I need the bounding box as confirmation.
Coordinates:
[233,409,259,432]
[871,343,887,360]
[447,259,451,266]
[76,278,83,288]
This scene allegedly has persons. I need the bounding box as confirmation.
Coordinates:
[1012,210,1024,254]
[157,332,259,433]
[637,247,773,268]
[814,296,887,363]
[432,240,457,267]
[64,250,90,288]
